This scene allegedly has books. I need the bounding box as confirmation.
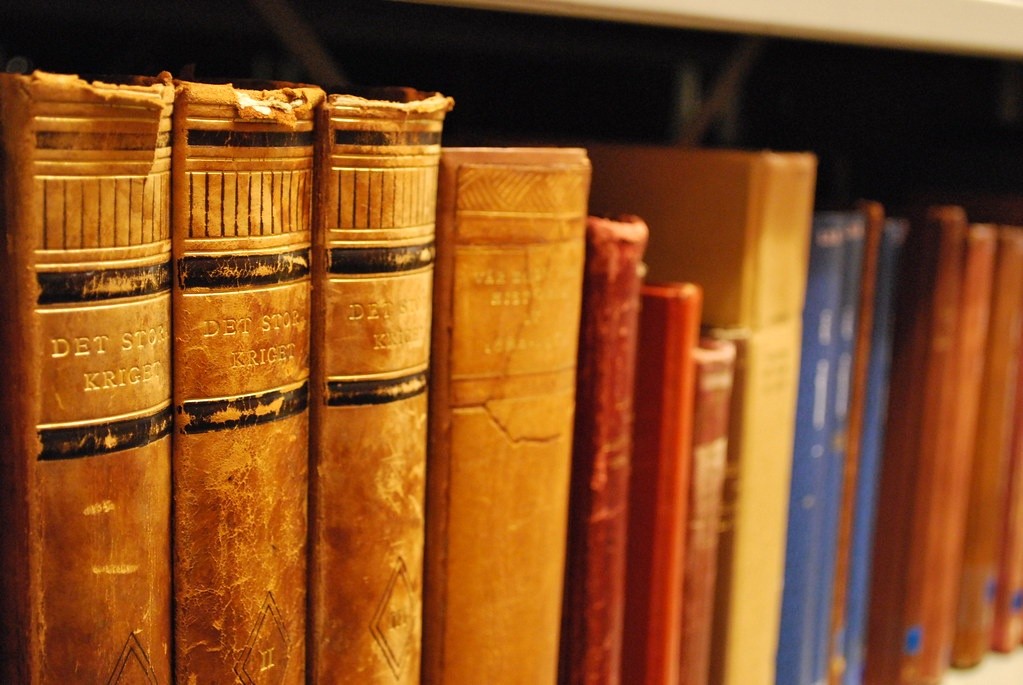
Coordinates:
[0,69,1023,685]
